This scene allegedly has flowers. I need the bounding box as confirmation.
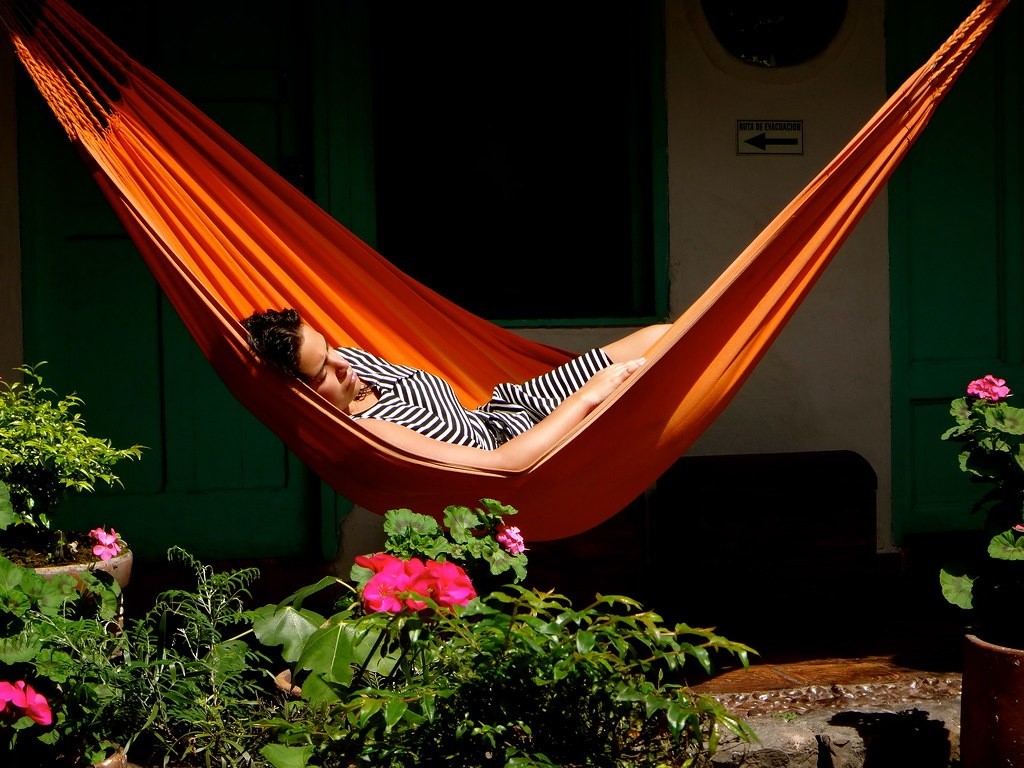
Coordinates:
[0,527,131,768]
[936,374,1024,649]
[250,495,528,766]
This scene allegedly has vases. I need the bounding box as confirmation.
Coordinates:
[36,739,128,768]
[960,633,1023,768]
[270,665,383,713]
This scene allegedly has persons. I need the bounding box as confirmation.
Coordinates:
[238,308,672,472]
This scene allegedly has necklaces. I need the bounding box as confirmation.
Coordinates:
[353,385,373,401]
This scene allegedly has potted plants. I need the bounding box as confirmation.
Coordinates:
[0,360,153,666]
